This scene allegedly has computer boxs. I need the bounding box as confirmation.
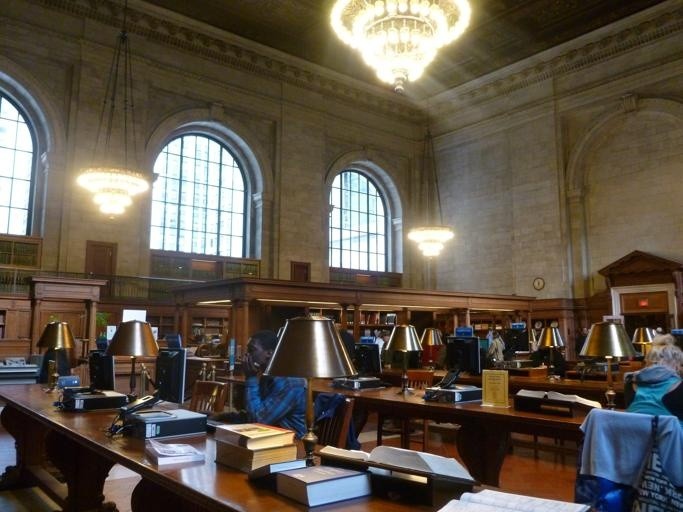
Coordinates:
[333,377,380,390]
[592,361,618,371]
[496,360,534,369]
[123,408,207,440]
[64,387,126,410]
[426,385,482,403]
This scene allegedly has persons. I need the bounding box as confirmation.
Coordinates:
[623,333,683,429]
[485,331,505,362]
[198,329,308,439]
[376,330,390,355]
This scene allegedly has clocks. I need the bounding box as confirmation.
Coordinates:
[533,277,545,290]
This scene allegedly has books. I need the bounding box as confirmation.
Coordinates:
[248,458,307,482]
[276,465,373,507]
[437,488,591,512]
[214,422,295,450]
[144,438,205,466]
[214,439,297,474]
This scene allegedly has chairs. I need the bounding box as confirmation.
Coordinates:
[0,350,683,512]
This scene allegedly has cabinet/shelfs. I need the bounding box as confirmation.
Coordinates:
[0,233,537,391]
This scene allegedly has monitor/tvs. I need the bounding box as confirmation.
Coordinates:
[64,349,115,393]
[504,328,529,361]
[122,347,187,413]
[166,335,182,350]
[347,343,382,380]
[432,336,480,388]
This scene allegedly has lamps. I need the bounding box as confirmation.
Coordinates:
[385,324,424,397]
[537,327,566,375]
[406,94,454,257]
[106,319,160,404]
[263,317,359,466]
[74,1,150,219]
[632,326,657,360]
[36,321,77,390]
[420,328,444,371]
[579,322,638,411]
[328,0,473,89]
